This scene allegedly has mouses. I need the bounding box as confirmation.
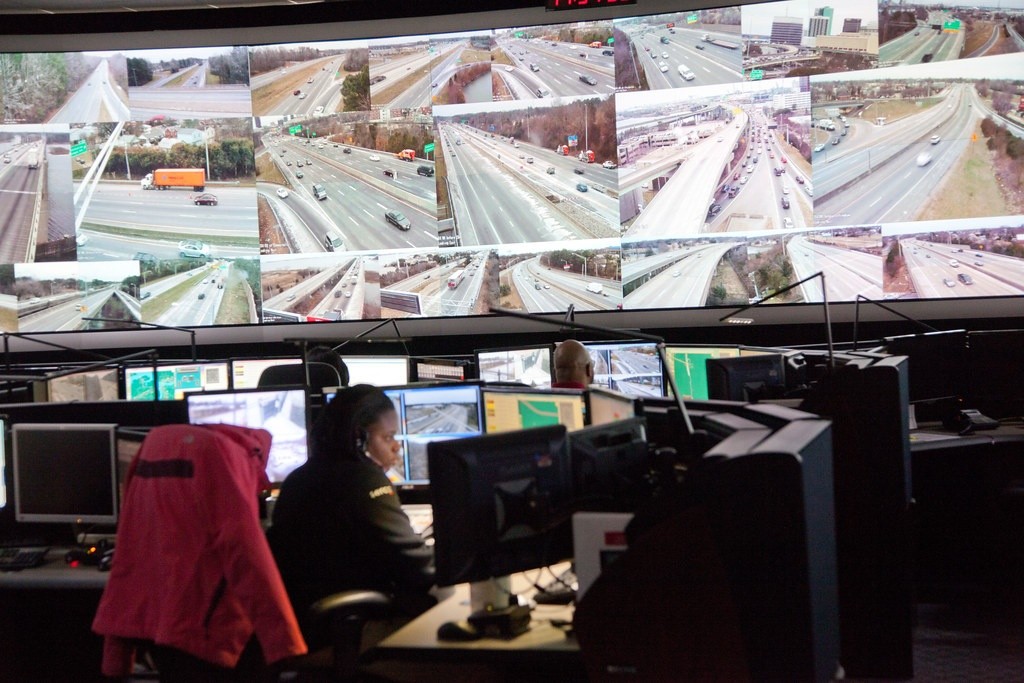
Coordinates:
[437,619,483,640]
[64,550,84,565]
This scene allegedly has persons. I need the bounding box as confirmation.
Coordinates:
[266,383,437,683]
[303,346,350,386]
[552,340,594,388]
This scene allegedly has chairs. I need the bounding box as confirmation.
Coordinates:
[97,421,390,683]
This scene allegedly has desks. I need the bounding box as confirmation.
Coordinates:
[1,498,588,683]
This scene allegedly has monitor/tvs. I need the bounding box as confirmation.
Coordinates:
[0,329,1024,590]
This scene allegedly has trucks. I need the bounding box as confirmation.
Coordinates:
[661,36,670,45]
[678,65,695,80]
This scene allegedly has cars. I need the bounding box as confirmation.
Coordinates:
[646,46,669,73]
[194,193,217,206]
[173,240,213,257]
[275,148,327,200]
[762,123,788,163]
[325,234,347,254]
[781,185,793,228]
[293,77,324,117]
[342,148,434,178]
[386,210,410,230]
[774,166,786,176]
[517,154,616,192]
[812,112,850,151]
[912,242,984,286]
[796,176,813,197]
[708,124,761,216]
[508,42,615,98]
[931,135,941,144]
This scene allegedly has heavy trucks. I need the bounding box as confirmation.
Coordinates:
[141,168,204,192]
[701,33,739,49]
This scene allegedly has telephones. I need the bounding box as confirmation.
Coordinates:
[941,408,1000,435]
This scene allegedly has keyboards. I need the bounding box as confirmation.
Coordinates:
[0,546,50,569]
[534,568,578,605]
